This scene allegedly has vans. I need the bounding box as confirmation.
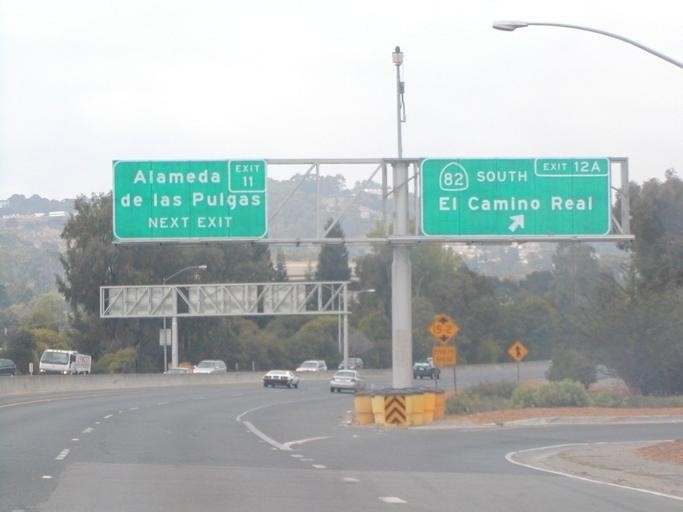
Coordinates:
[413,358,440,379]
[0,358,16,376]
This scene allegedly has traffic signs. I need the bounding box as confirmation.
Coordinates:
[419,156,612,236]
[112,159,269,239]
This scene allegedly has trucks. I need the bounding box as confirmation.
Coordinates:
[39,349,92,375]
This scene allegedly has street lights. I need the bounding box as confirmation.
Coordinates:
[162,265,208,374]
[338,288,376,351]
[492,20,683,69]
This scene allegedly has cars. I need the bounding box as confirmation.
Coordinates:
[262,357,366,393]
[193,359,227,374]
